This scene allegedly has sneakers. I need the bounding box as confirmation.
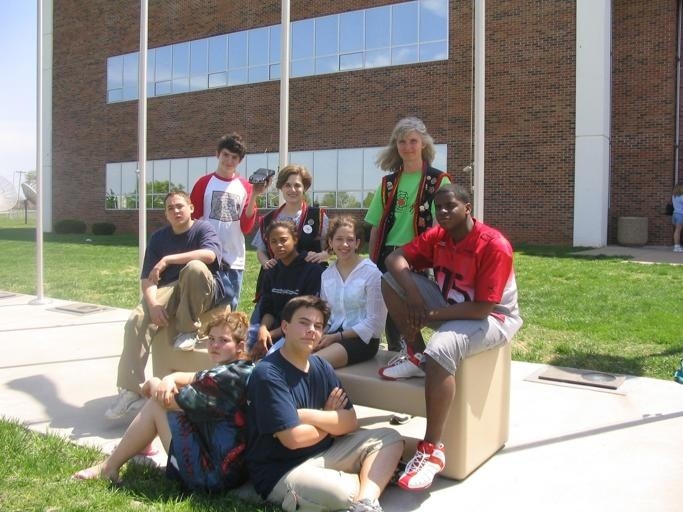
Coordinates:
[387,466,407,487]
[345,495,385,512]
[389,411,414,425]
[102,386,145,421]
[394,438,447,493]
[171,329,200,353]
[376,348,432,383]
[672,244,683,253]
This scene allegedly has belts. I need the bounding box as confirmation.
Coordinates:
[379,243,406,254]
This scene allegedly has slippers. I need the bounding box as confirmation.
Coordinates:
[70,463,126,486]
[109,437,160,460]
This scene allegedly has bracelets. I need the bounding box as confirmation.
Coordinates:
[338,325,346,340]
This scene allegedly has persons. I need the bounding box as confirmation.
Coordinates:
[249,163,330,302]
[245,296,406,512]
[311,215,389,369]
[190,132,273,313]
[364,116,452,429]
[71,309,256,493]
[104,186,223,420]
[247,218,324,361]
[378,182,524,490]
[671,185,683,251]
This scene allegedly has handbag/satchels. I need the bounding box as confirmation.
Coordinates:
[664,193,676,216]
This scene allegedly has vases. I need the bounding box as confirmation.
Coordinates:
[617,215,649,247]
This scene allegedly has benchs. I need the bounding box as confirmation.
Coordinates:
[149,318,511,483]
